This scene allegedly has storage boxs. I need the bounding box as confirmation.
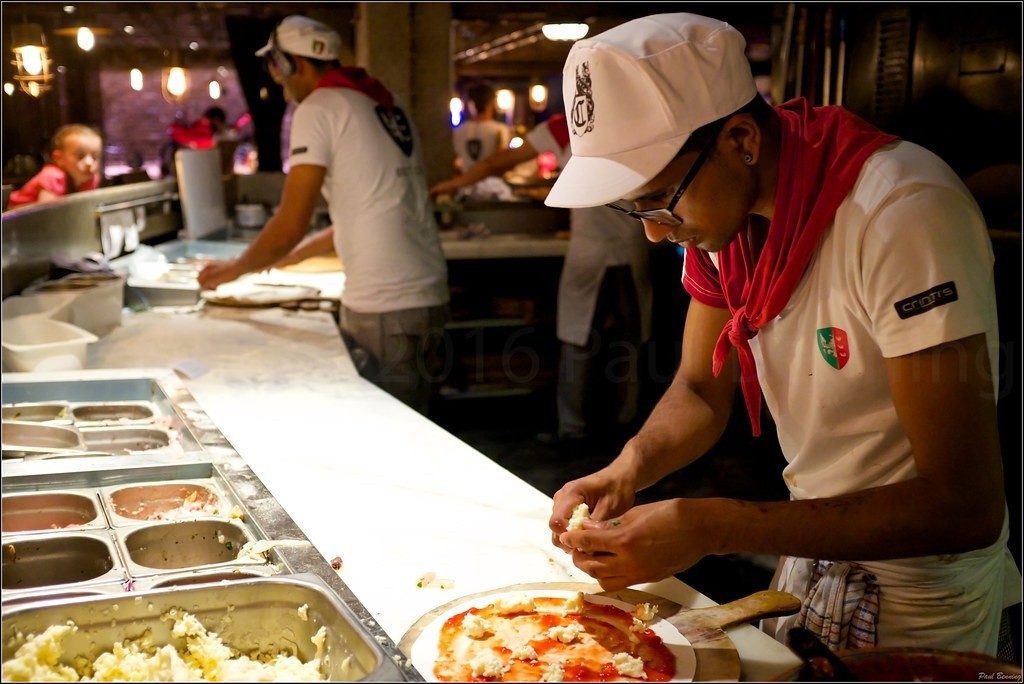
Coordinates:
[1,276,124,374]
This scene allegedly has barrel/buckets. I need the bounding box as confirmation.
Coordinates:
[774,648,1022,682]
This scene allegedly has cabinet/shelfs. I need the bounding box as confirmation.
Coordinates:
[446,296,538,402]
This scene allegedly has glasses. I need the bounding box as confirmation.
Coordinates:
[603,118,729,227]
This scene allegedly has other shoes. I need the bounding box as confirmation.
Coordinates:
[535,431,587,449]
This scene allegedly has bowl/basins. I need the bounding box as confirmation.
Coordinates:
[235,203,266,227]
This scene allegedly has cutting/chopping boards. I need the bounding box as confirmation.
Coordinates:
[393,581,802,683]
[217,281,340,310]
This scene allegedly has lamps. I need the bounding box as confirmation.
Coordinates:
[9,12,55,98]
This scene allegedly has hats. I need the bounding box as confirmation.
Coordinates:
[542,9,764,209]
[254,14,343,63]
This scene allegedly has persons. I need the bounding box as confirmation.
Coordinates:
[544,12,1015,647]
[454,85,511,199]
[198,18,449,409]
[6,123,101,206]
[160,108,226,177]
[429,94,653,436]
